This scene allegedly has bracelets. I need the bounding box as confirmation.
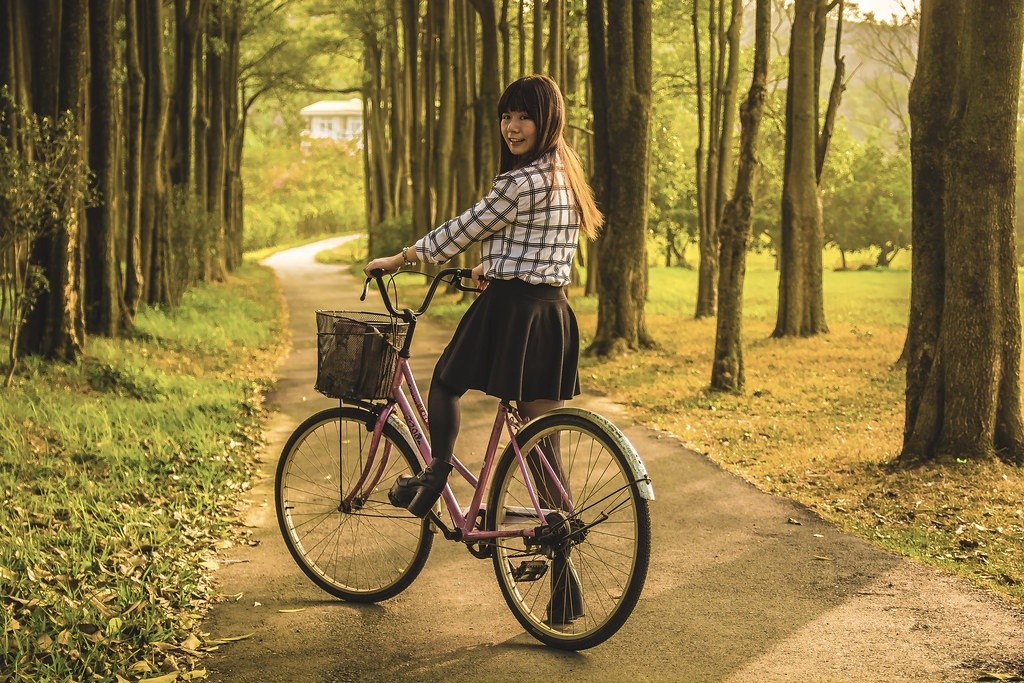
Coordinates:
[402,247,415,266]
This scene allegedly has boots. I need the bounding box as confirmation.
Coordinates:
[390,457,454,519]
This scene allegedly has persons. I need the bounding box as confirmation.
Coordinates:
[363,75,603,623]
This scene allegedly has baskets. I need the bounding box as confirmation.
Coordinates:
[315,310,410,398]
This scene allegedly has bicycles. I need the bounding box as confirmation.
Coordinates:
[273,266,657,653]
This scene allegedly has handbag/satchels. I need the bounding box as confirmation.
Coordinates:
[317,319,407,398]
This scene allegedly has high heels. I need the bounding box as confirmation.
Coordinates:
[546,558,584,623]
